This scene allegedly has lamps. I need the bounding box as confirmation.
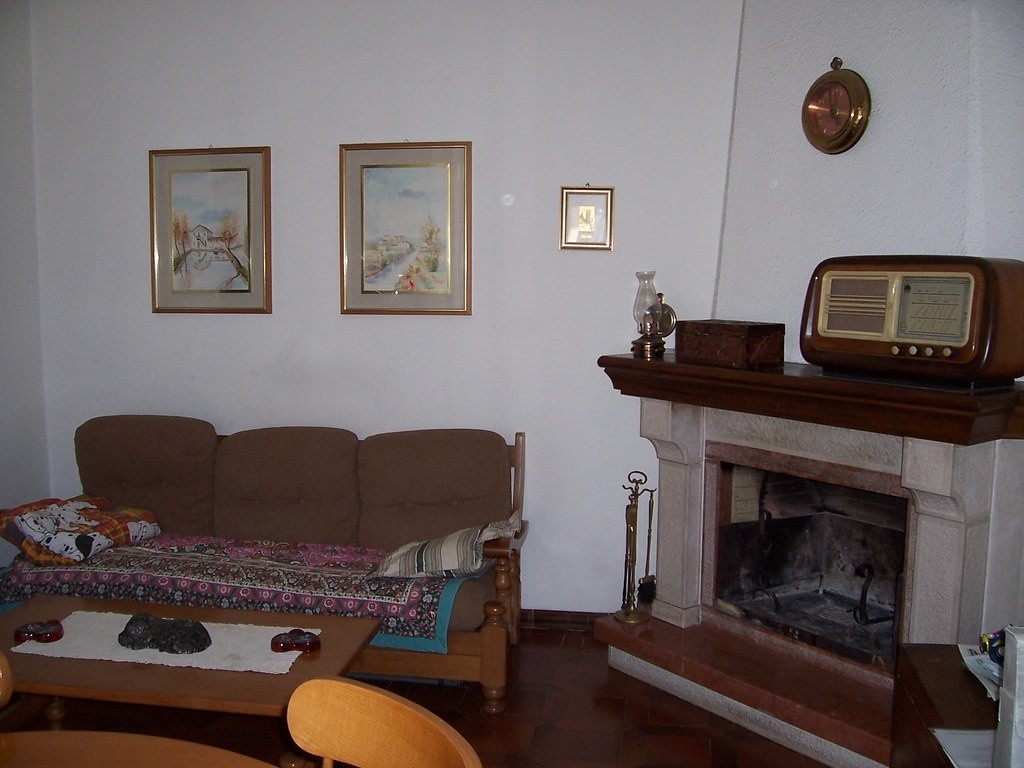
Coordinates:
[631,271,666,361]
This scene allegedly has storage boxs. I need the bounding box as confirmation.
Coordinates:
[675,319,785,369]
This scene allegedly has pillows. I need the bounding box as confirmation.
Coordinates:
[364,523,489,582]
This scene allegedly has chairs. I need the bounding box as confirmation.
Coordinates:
[287,674,482,768]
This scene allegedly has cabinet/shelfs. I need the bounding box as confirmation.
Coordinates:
[891,644,1000,768]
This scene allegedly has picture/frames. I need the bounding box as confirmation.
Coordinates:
[338,141,472,316]
[149,146,273,315]
[559,185,616,250]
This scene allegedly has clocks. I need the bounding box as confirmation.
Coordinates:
[801,57,871,155]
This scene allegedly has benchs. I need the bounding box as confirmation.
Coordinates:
[0,415,529,716]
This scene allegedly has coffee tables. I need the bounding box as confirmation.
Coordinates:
[0,593,382,731]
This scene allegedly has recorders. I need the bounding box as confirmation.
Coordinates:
[799,255,1024,390]
